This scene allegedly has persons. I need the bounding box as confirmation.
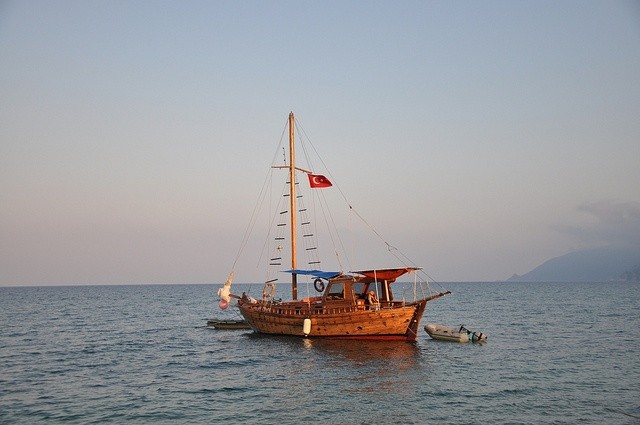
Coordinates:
[369,291,380,307]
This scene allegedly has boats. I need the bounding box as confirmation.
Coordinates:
[423,321,489,344]
[207,318,252,331]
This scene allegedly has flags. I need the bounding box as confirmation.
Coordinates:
[307,174,332,188]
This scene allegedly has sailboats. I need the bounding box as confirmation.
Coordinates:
[216,110,452,343]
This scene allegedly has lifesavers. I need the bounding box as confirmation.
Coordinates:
[314,279,324,292]
[263,284,275,297]
